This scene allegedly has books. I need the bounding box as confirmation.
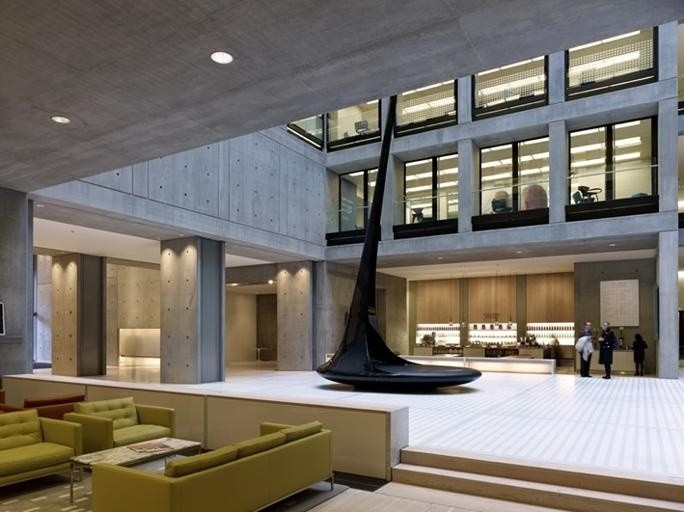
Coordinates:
[128,441,174,452]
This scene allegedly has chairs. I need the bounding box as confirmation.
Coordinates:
[411,208,424,222]
[0,389,176,486]
[573,185,603,202]
[491,198,512,213]
[354,120,372,135]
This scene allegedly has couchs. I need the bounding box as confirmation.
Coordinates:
[91,422,335,512]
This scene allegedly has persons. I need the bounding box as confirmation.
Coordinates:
[597,320,618,379]
[574,320,592,377]
[618,336,629,349]
[630,333,648,376]
